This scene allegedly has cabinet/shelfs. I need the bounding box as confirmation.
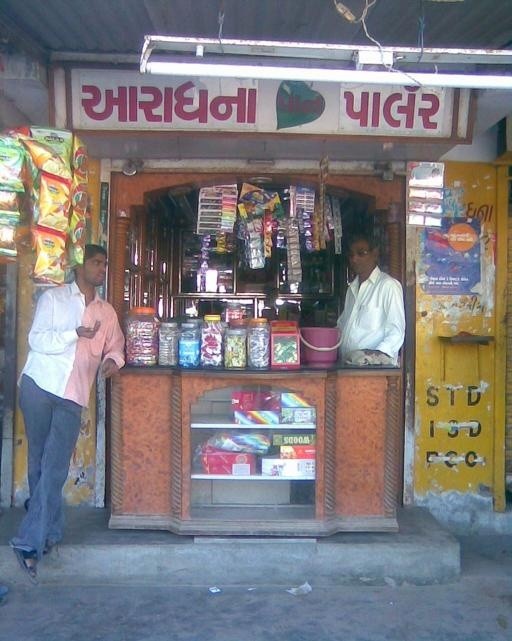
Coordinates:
[107,367,405,538]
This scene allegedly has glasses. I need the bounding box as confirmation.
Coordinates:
[347,248,372,258]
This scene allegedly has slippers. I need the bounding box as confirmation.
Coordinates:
[8,498,50,586]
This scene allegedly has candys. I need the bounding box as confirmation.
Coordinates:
[125,322,297,370]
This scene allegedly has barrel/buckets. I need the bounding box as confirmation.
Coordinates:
[124,305,160,369]
[299,326,344,368]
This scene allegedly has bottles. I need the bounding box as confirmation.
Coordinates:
[126,308,269,374]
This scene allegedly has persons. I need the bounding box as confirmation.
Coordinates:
[337,234,405,367]
[9,244,125,584]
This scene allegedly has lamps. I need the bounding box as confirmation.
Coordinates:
[140,33,512,91]
